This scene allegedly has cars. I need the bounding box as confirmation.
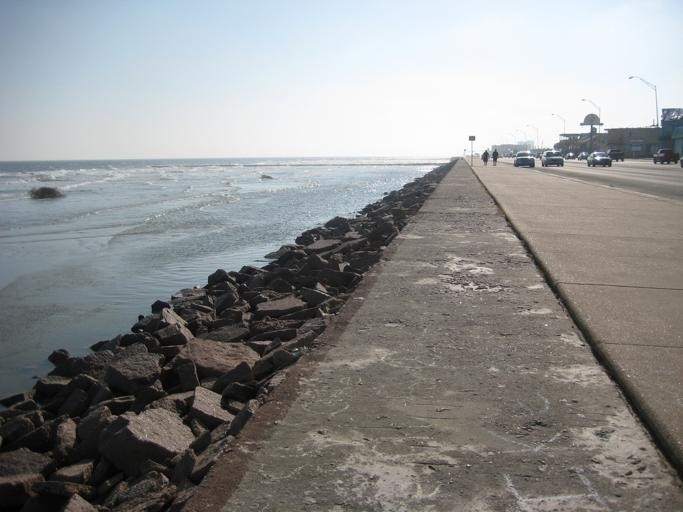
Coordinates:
[565,153,576,160]
[586,151,612,166]
[513,151,535,168]
[541,150,563,167]
[578,151,588,160]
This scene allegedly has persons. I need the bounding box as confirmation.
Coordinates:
[480,150,488,166]
[491,149,497,167]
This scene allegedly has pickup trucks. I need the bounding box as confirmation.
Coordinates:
[607,149,624,162]
[653,148,679,165]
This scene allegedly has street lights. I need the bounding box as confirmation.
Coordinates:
[552,114,566,137]
[581,98,600,150]
[628,76,659,129]
